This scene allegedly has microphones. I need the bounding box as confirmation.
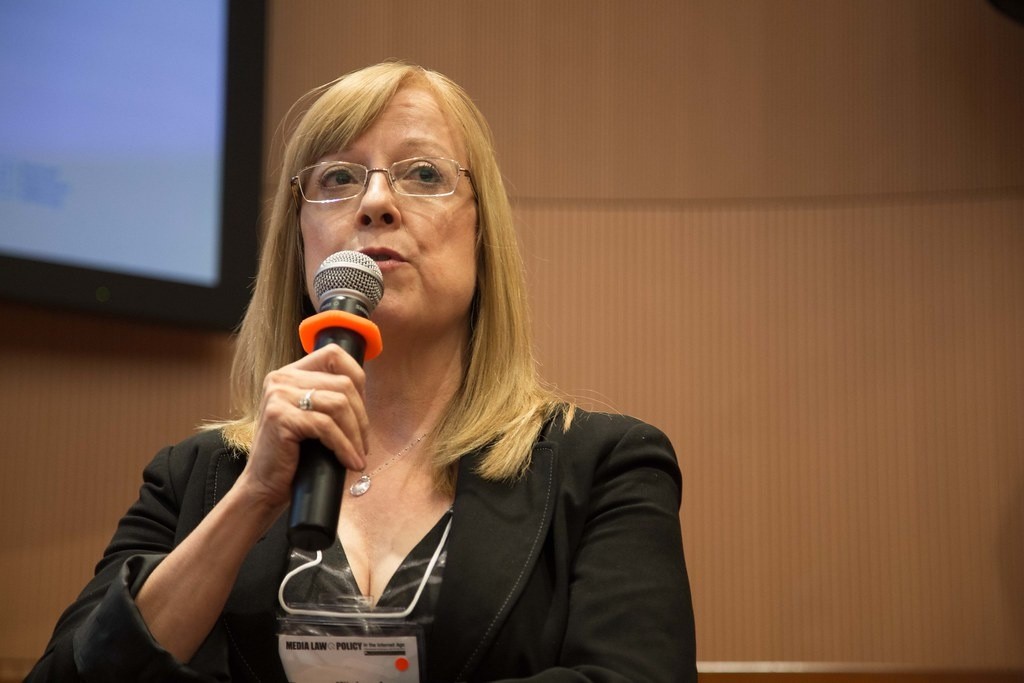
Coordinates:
[285,250,384,553]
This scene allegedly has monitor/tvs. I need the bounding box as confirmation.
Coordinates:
[0,0,267,332]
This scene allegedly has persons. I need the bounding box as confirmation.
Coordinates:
[28,62,698,683]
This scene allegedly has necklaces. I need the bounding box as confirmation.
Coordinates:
[349,432,427,496]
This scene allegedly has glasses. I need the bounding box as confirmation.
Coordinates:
[290,154,474,221]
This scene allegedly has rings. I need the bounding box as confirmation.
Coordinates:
[299,388,314,409]
